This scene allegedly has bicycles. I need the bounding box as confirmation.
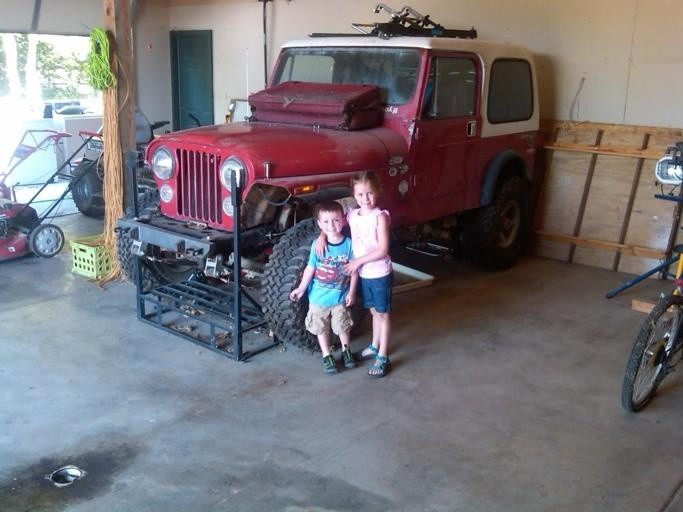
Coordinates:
[604,140,682,414]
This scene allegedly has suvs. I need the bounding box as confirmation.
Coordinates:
[111,6,545,358]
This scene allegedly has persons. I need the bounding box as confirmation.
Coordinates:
[312,171,394,378]
[289,199,359,375]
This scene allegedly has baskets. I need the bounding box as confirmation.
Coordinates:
[69,233,114,281]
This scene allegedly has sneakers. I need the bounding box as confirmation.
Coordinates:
[321,354,338,374]
[340,344,357,369]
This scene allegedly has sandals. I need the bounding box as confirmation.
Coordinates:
[366,352,391,379]
[352,343,378,361]
